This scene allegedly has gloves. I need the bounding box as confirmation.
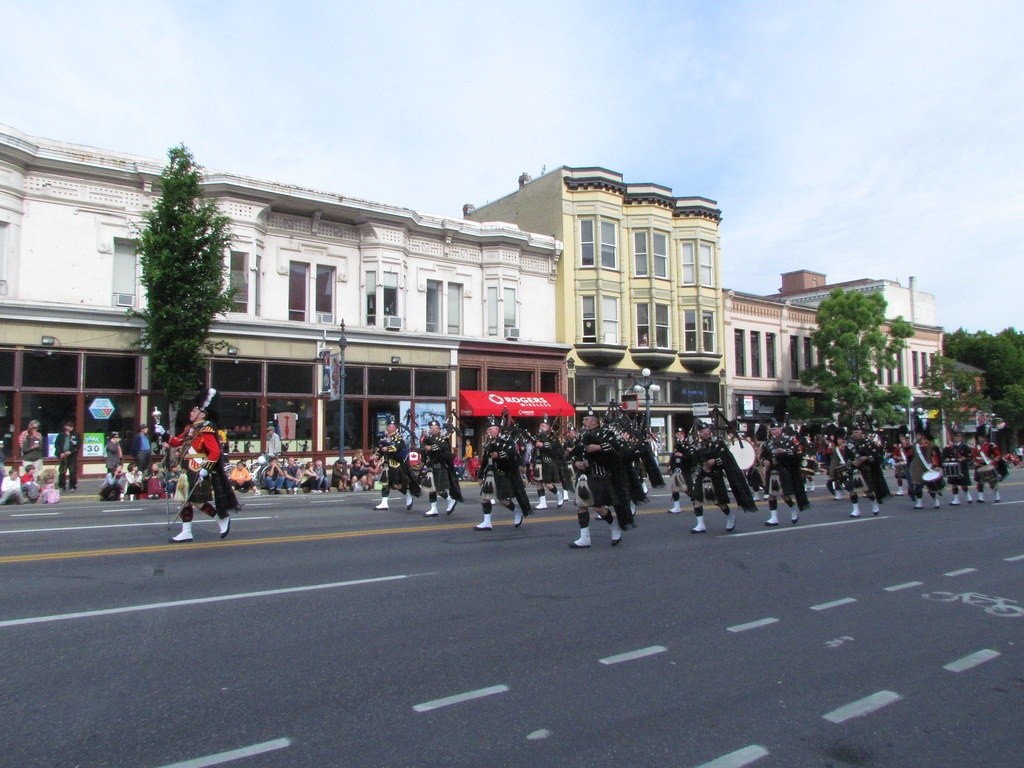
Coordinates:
[154,424,165,435]
[199,467,208,477]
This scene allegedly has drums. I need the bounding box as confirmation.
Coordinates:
[800,456,816,473]
[942,462,962,477]
[724,438,755,470]
[922,470,948,493]
[977,465,1000,484]
[894,460,908,479]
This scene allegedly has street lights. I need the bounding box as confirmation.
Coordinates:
[900,397,923,441]
[634,367,660,428]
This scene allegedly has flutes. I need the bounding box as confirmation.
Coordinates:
[424,407,468,464]
[485,408,532,476]
[376,409,420,462]
[575,399,633,471]
[534,415,572,461]
[773,411,813,469]
[632,413,660,448]
[566,426,586,455]
[674,419,700,457]
[698,405,744,473]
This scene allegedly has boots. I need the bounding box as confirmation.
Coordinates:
[213,513,231,538]
[169,521,193,542]
[373,480,1001,547]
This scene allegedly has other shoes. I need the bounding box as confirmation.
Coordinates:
[253,487,372,498]
[148,493,161,499]
[70,488,74,493]
[59,488,66,494]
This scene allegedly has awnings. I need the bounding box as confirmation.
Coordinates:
[458,389,575,418]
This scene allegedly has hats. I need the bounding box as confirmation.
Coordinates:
[385,400,991,437]
[64,421,75,426]
[139,424,150,429]
[195,385,221,418]
[267,426,275,430]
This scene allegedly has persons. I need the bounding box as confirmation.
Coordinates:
[331,460,349,491]
[284,456,304,495]
[98,460,182,502]
[132,423,151,469]
[350,449,379,492]
[299,459,330,494]
[265,426,282,463]
[666,409,1024,534]
[372,400,664,548]
[154,405,244,542]
[262,457,285,495]
[106,432,123,471]
[0,441,62,506]
[55,420,83,494]
[18,419,43,476]
[228,461,262,497]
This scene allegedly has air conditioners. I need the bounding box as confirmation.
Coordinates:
[117,294,134,307]
[318,313,333,324]
[383,315,401,330]
[504,327,520,340]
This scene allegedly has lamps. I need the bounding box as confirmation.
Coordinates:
[42,336,55,346]
[392,357,400,363]
[228,347,238,355]
